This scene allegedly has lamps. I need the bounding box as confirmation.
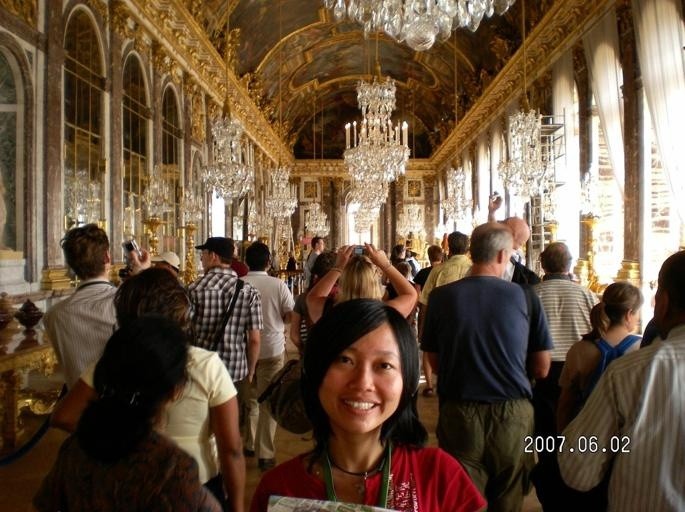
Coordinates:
[395,35,474,235]
[319,1,515,50]
[344,32,411,233]
[497,1,554,199]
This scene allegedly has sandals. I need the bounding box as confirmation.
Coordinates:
[423,388,434,397]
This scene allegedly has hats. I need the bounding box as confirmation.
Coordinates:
[195,238,234,257]
[150,251,180,269]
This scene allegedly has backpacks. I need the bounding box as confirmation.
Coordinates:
[510,256,540,286]
[580,334,645,400]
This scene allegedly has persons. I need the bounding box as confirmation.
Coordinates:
[33,317,224,511]
[40,224,293,467]
[529,242,597,511]
[414,216,667,445]
[286,236,418,320]
[248,299,489,512]
[419,222,555,512]
[555,252,685,512]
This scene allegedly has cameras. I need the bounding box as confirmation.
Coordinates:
[121,239,142,258]
[354,247,364,255]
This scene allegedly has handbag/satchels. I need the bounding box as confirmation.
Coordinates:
[257,360,313,434]
